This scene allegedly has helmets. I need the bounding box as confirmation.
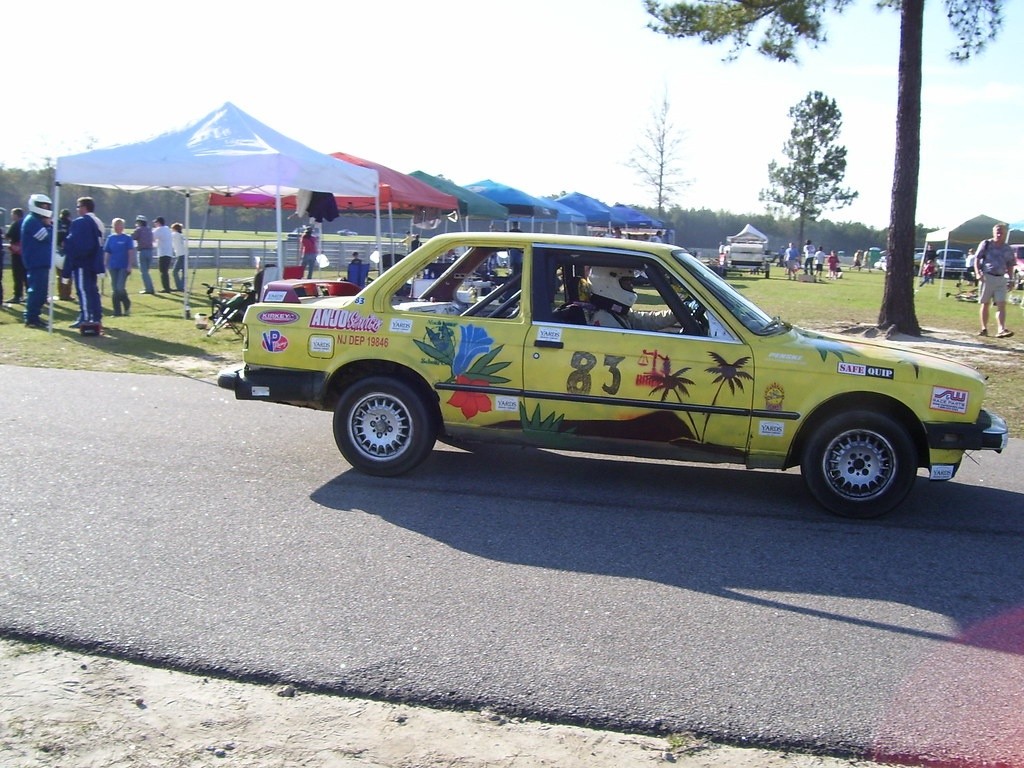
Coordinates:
[28,195,53,217]
[587,261,649,307]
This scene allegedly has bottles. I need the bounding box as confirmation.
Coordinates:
[186,304,191,320]
[194,314,207,329]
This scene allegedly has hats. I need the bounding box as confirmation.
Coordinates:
[136,215,146,221]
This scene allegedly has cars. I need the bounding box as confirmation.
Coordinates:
[913,248,924,276]
[1004,244,1024,290]
[934,249,967,278]
[336,229,357,235]
[288,225,319,240]
[219,231,1007,520]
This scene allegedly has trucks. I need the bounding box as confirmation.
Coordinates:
[719,241,770,278]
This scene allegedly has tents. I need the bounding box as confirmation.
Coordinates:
[48,102,665,310]
[913,215,1024,299]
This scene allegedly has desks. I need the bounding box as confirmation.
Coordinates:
[413,279,437,299]
[219,276,255,284]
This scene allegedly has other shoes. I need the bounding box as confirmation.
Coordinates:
[980,329,987,337]
[158,289,169,293]
[124,302,131,315]
[69,320,82,327]
[172,288,184,292]
[5,295,20,303]
[139,290,150,294]
[995,329,1013,338]
[25,317,49,328]
[60,295,75,300]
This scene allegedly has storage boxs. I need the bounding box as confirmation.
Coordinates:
[457,291,470,303]
[218,291,237,298]
[80,322,101,336]
[798,274,816,283]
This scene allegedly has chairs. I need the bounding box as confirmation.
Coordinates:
[284,265,305,281]
[555,302,588,326]
[202,264,278,340]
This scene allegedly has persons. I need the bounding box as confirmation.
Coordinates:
[580,264,703,334]
[648,230,664,243]
[411,233,425,255]
[854,244,978,287]
[776,239,840,283]
[719,242,725,253]
[605,228,612,238]
[295,228,321,279]
[643,233,650,241]
[403,231,413,257]
[974,223,1014,337]
[508,222,524,233]
[613,227,627,239]
[0,193,187,329]
[350,252,363,265]
